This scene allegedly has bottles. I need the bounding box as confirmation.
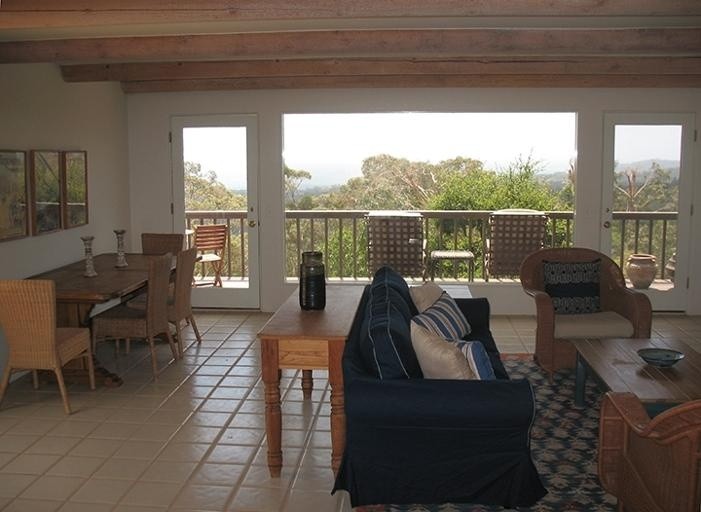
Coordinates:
[300,252,326,312]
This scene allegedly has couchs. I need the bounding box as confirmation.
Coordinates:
[328,263,551,510]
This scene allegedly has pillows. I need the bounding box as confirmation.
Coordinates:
[400,280,497,382]
[540,255,605,316]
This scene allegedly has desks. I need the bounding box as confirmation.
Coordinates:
[254,270,481,482]
[426,247,478,284]
[25,253,202,386]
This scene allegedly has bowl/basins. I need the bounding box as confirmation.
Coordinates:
[638,348,687,368]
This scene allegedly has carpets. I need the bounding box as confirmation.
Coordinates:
[347,350,682,512]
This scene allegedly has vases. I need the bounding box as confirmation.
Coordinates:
[298,250,327,311]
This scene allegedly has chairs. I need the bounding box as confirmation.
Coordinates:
[480,207,554,286]
[186,225,227,287]
[515,244,653,391]
[595,390,701,512]
[121,234,184,343]
[93,252,179,377]
[362,210,432,284]
[127,249,202,359]
[2,279,96,413]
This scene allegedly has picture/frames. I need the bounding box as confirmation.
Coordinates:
[61,149,93,231]
[1,148,28,245]
[28,147,66,238]
